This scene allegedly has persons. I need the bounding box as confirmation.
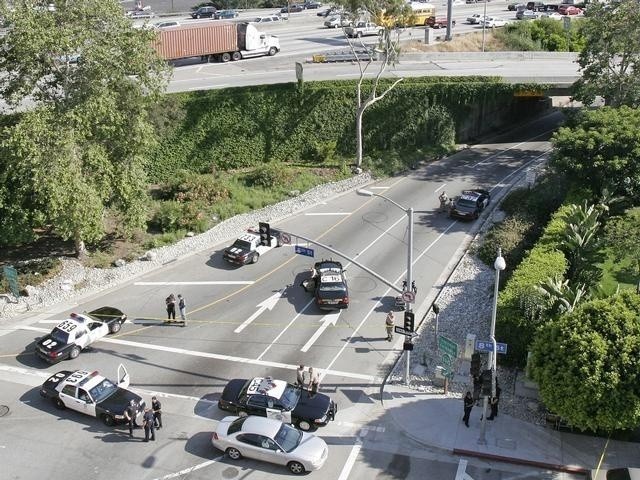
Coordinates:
[384,310,396,342]
[447,198,454,219]
[487,396,500,421]
[141,407,156,443]
[151,396,163,431]
[461,391,475,428]
[178,294,187,327]
[123,399,142,437]
[165,293,177,323]
[296,364,306,389]
[307,367,321,393]
[437,191,448,215]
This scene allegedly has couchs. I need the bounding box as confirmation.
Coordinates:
[406,316,411,332]
[260,227,268,247]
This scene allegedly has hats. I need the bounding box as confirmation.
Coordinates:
[223,225,285,268]
[35,306,125,365]
[301,258,349,310]
[218,377,338,434]
[40,362,145,426]
[211,415,329,475]
[0,2,237,83]
[449,189,490,221]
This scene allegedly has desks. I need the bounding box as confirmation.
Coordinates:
[473,244,507,444]
[355,187,412,384]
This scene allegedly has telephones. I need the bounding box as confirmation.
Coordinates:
[395,327,418,339]
[477,341,506,353]
